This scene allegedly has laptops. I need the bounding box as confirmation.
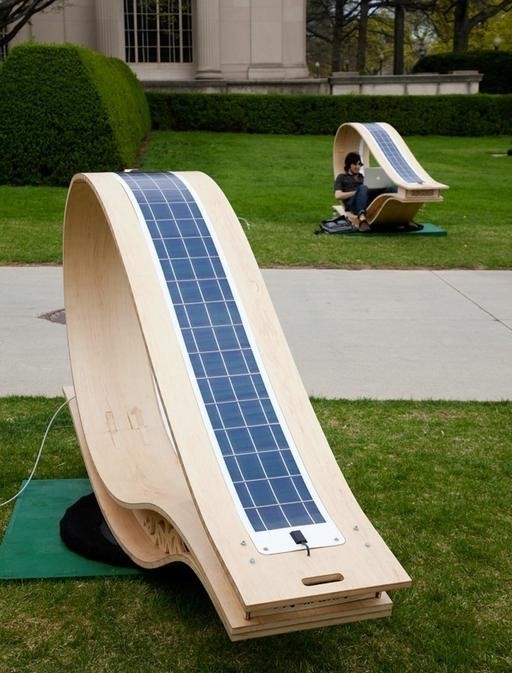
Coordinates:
[363,167,393,190]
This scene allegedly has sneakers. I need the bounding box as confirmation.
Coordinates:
[359,220,371,231]
[409,220,424,230]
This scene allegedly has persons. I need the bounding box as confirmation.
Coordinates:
[335,151,425,232]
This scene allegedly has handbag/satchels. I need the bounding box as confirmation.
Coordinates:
[319,215,353,234]
